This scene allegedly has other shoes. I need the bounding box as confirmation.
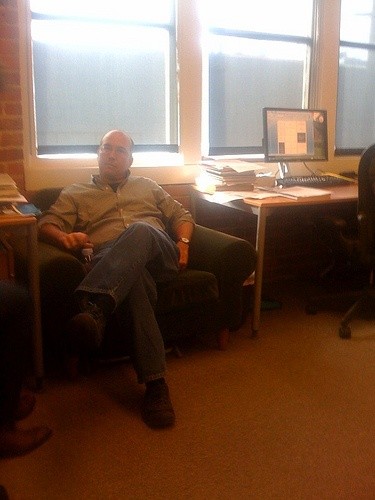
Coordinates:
[74,295,109,355]
[142,379,175,428]
[17,387,38,418]
[1,426,53,459]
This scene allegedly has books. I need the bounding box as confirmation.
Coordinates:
[197,160,256,192]
[0,172,28,203]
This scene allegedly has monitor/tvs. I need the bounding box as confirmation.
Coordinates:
[261,107,330,177]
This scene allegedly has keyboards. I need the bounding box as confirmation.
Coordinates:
[276,175,347,187]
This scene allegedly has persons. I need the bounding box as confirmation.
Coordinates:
[35,129,196,429]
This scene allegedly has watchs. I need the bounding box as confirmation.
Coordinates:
[177,238,192,245]
